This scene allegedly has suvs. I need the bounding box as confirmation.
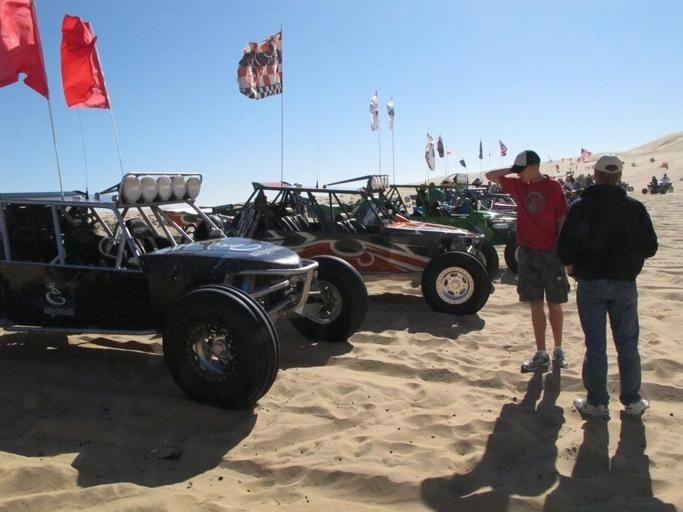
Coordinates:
[0,174,322,411]
[642,182,673,195]
[298,173,633,282]
[160,174,501,314]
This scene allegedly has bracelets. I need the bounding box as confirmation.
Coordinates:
[567,273,575,276]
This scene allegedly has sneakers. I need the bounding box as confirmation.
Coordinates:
[575,399,609,418]
[521,352,550,372]
[625,399,649,415]
[553,350,567,368]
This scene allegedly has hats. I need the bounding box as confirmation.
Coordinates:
[592,155,624,174]
[510,151,539,172]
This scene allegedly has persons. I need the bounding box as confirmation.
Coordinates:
[555,155,657,418]
[485,150,569,370]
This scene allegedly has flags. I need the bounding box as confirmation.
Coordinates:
[368,96,378,131]
[580,148,593,162]
[237,32,282,99]
[499,141,506,156]
[386,101,394,132]
[59,14,109,109]
[0,1,48,102]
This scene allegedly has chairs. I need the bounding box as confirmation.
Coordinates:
[123,219,163,251]
[336,213,367,233]
[280,207,312,230]
